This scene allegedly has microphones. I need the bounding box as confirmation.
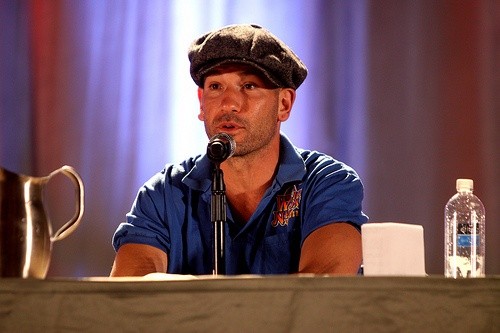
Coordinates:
[205,131,237,162]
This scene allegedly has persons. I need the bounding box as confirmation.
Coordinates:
[109,24,371,278]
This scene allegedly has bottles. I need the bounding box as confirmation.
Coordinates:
[443,178,486,278]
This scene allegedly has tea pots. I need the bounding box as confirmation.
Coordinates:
[1,164,85,279]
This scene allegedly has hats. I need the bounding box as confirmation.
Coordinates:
[188,23,310,89]
